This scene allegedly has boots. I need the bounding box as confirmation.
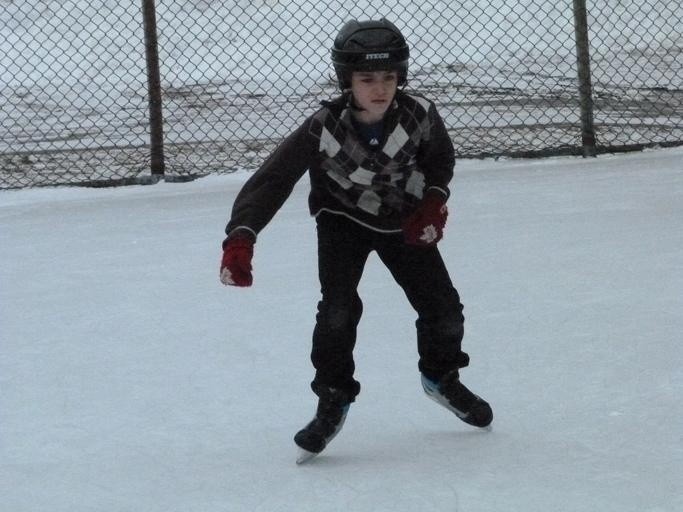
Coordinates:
[294,385,351,453]
[421,369,493,427]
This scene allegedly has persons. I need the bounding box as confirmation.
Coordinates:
[219,18,493,453]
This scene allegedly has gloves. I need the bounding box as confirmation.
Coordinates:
[400,192,448,249]
[220,233,253,287]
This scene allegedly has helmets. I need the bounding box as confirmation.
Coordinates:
[331,18,410,89]
